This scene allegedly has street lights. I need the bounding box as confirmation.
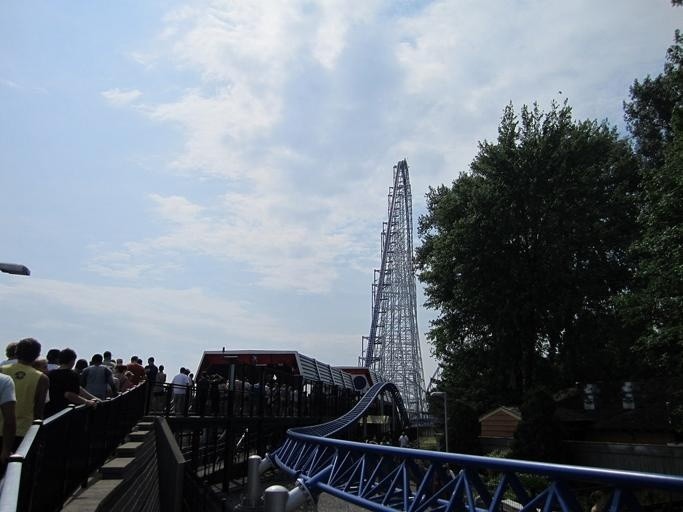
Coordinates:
[430,391,449,452]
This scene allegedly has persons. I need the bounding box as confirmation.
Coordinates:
[368,438,376,444]
[1,334,158,477]
[152,365,166,411]
[398,431,409,447]
[169,367,298,416]
[585,489,609,512]
